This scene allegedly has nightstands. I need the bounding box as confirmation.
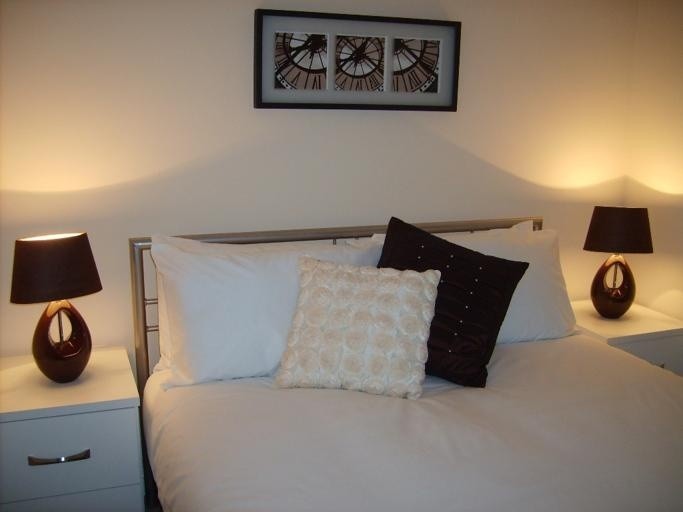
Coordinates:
[0,346,146,511]
[569,300,682,378]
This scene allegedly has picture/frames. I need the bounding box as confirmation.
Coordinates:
[252,10,461,112]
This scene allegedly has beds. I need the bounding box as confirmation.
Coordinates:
[128,218,680,511]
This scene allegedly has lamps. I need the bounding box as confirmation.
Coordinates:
[9,233,102,383]
[582,205,654,320]
